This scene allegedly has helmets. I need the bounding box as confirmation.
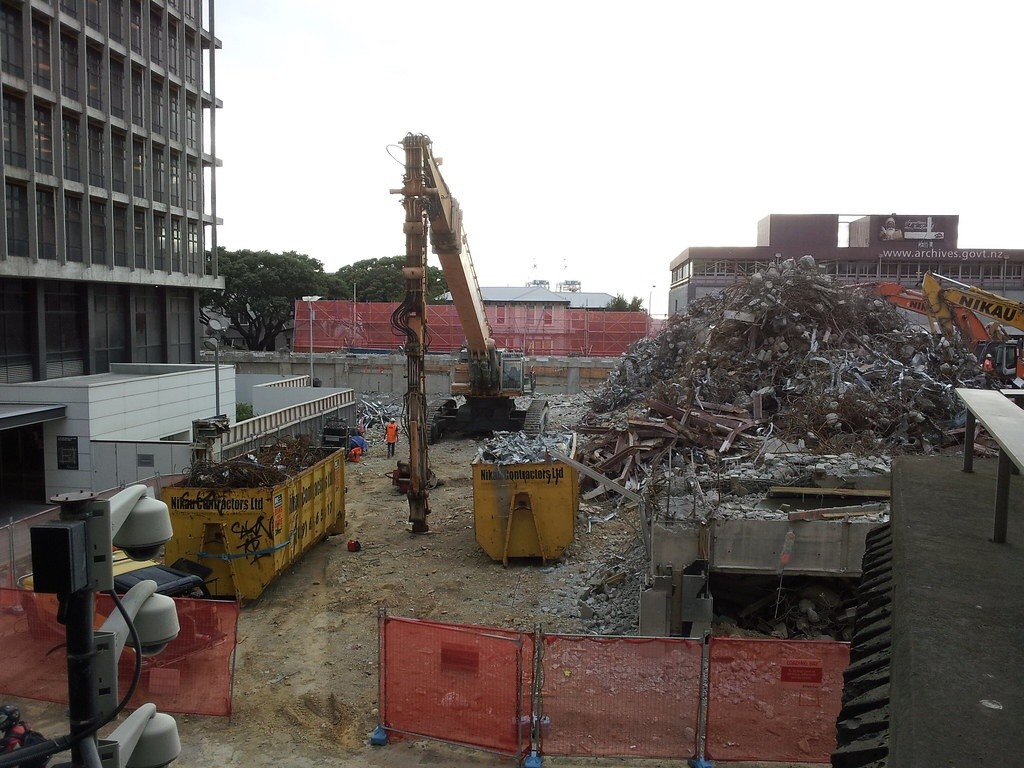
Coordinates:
[389,418,396,422]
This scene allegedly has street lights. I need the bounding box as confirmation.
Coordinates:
[302,295,322,387]
[204,318,219,417]
[648,284,656,337]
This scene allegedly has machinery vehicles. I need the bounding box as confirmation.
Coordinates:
[388,131,548,535]
[920,270,1024,365]
[839,280,1024,385]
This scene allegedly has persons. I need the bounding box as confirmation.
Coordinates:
[383,418,398,459]
[984,353,994,386]
